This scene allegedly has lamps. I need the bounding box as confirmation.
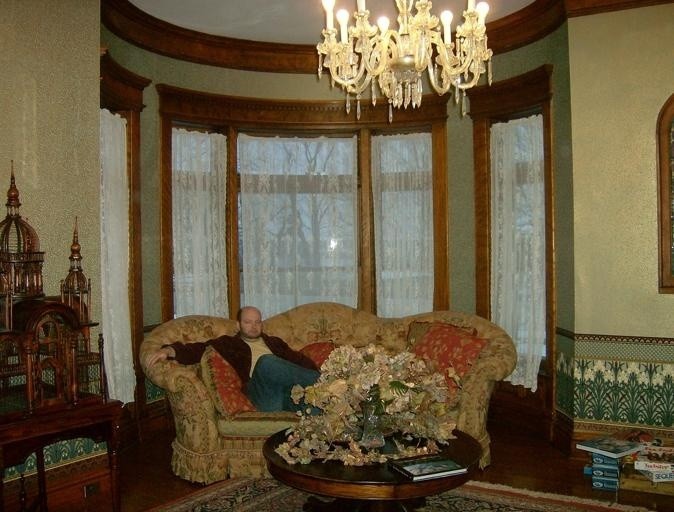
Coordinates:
[317,0,494,123]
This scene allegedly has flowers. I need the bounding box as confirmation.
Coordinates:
[274,343,462,468]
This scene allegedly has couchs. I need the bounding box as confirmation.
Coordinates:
[139,302,517,485]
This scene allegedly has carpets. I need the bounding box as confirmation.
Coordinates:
[152,471,650,512]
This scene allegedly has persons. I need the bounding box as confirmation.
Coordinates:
[142,305,324,418]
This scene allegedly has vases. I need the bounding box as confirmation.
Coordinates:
[358,401,385,448]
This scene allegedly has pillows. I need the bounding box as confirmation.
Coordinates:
[416,321,489,410]
[405,320,477,353]
[296,337,338,371]
[199,345,258,417]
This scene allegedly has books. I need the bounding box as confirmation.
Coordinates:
[389,454,470,481]
[638,470,674,482]
[633,445,674,471]
[576,436,644,459]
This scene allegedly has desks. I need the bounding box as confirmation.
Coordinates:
[0,381,124,512]
[262,425,482,512]
